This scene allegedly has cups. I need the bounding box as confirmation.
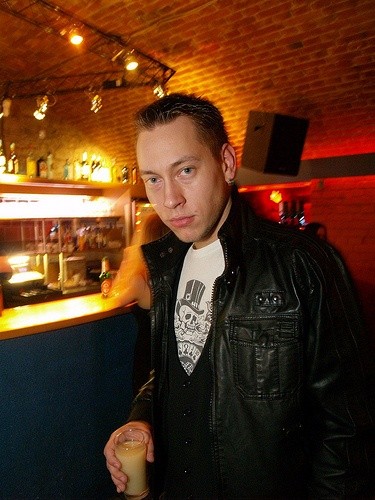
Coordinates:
[115,428,149,500]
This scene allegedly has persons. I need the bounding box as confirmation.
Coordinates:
[305,223,328,242]
[111,214,167,400]
[103,92,375,500]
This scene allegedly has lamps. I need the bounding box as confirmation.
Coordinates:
[33,98,49,120]
[68,23,85,46]
[89,94,103,113]
[112,49,140,72]
[153,85,172,99]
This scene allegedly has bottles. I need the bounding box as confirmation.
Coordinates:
[0,139,7,175]
[276,196,308,231]
[26,150,137,187]
[99,255,113,298]
[7,142,19,175]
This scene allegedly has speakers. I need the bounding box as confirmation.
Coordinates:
[240,110,309,177]
